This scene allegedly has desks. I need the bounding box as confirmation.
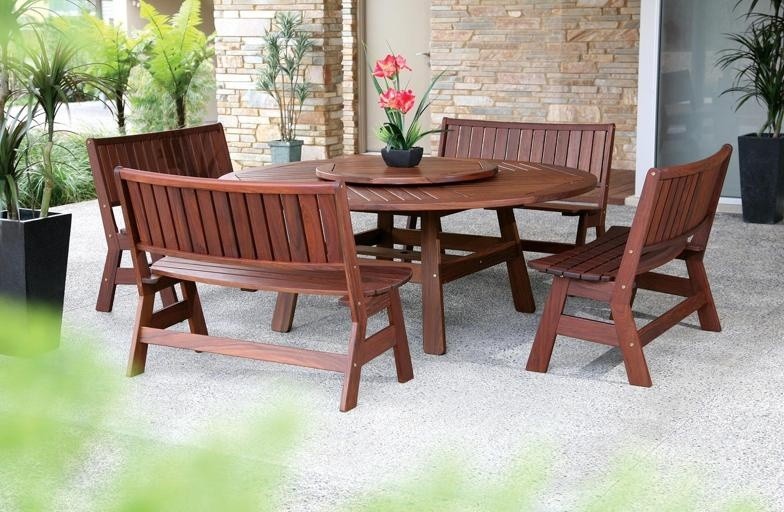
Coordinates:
[214,153,598,356]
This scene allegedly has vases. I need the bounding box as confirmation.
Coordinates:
[380,146,424,167]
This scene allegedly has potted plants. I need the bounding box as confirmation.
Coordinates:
[713,0,784,225]
[0,0,131,357]
[252,9,318,167]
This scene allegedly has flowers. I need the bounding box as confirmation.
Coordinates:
[356,35,449,147]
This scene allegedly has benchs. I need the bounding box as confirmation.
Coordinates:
[400,115,617,263]
[523,138,731,389]
[108,162,415,412]
[83,125,233,314]
